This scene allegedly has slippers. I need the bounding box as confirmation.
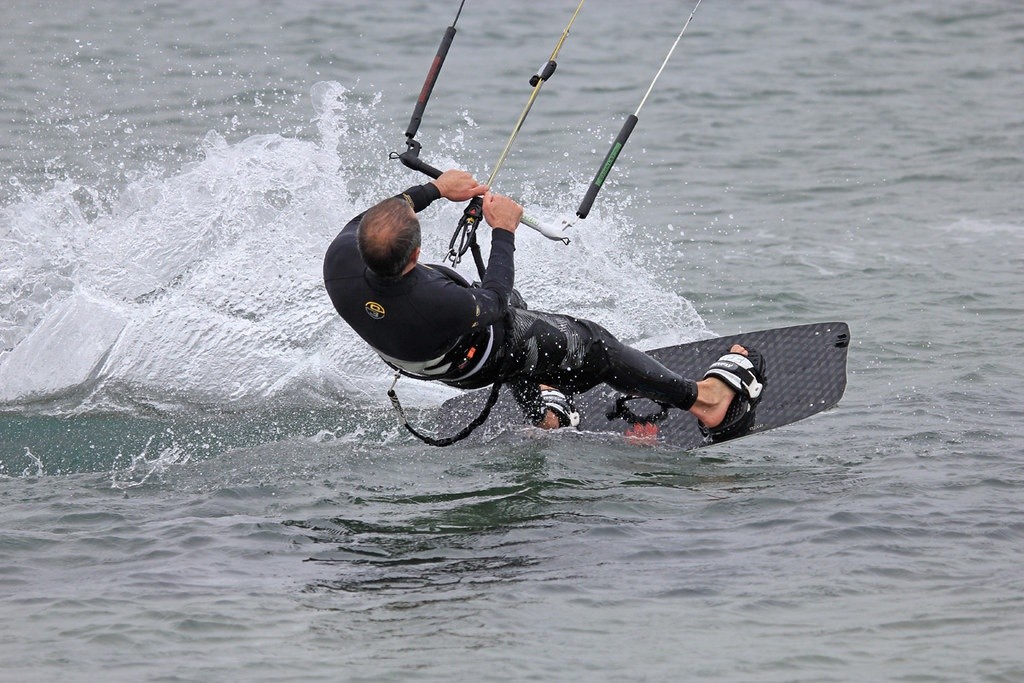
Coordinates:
[540,385,581,431]
[697,345,767,441]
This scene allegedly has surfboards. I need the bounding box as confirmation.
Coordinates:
[435,321,850,451]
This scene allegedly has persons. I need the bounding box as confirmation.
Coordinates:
[322,170,747,428]
